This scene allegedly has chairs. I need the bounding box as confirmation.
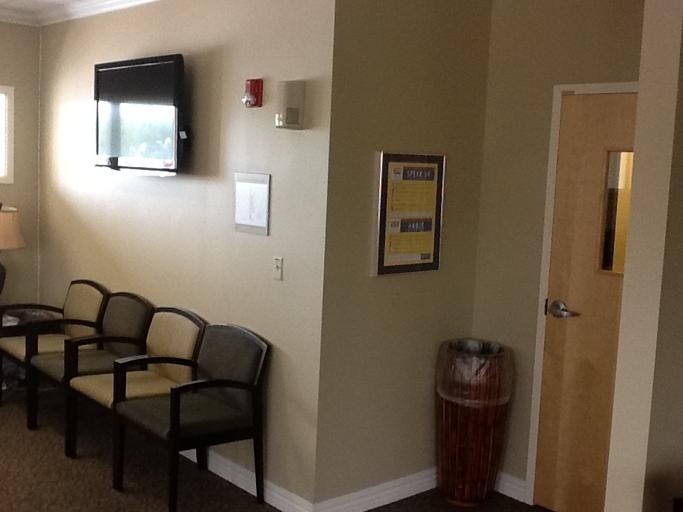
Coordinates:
[115,322,272,512]
[29,292,155,456]
[65,307,208,489]
[1,280,111,429]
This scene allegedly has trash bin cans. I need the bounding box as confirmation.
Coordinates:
[434,337,517,507]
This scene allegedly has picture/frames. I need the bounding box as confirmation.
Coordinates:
[376,149,445,275]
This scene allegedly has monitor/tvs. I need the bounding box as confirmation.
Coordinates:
[94,53,184,174]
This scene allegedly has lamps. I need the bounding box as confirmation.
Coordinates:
[1,202,28,293]
